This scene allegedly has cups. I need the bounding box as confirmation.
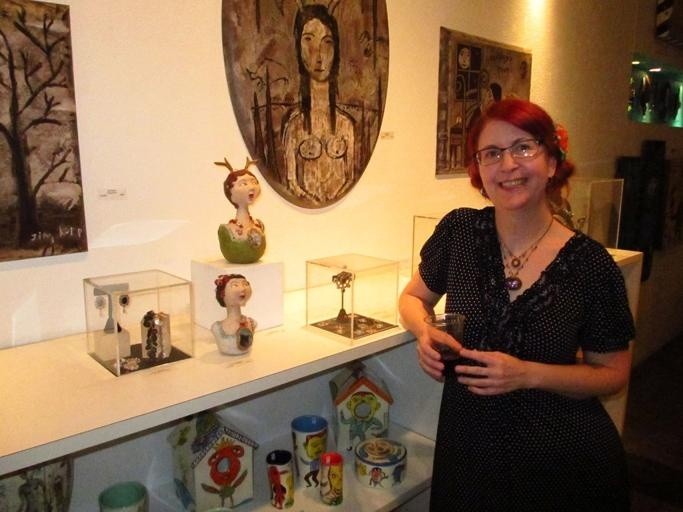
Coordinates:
[266,449,295,510]
[291,414,328,488]
[423,313,465,360]
[319,452,344,506]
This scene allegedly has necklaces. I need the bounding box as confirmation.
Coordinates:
[493,214,555,293]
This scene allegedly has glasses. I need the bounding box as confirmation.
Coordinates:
[474,139,547,166]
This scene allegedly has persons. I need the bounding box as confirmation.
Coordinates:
[398,99,639,510]
[465,82,503,134]
[212,153,269,265]
[208,272,258,354]
[456,44,472,69]
[279,6,358,207]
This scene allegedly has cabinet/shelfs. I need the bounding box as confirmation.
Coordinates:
[1,246,644,512]
